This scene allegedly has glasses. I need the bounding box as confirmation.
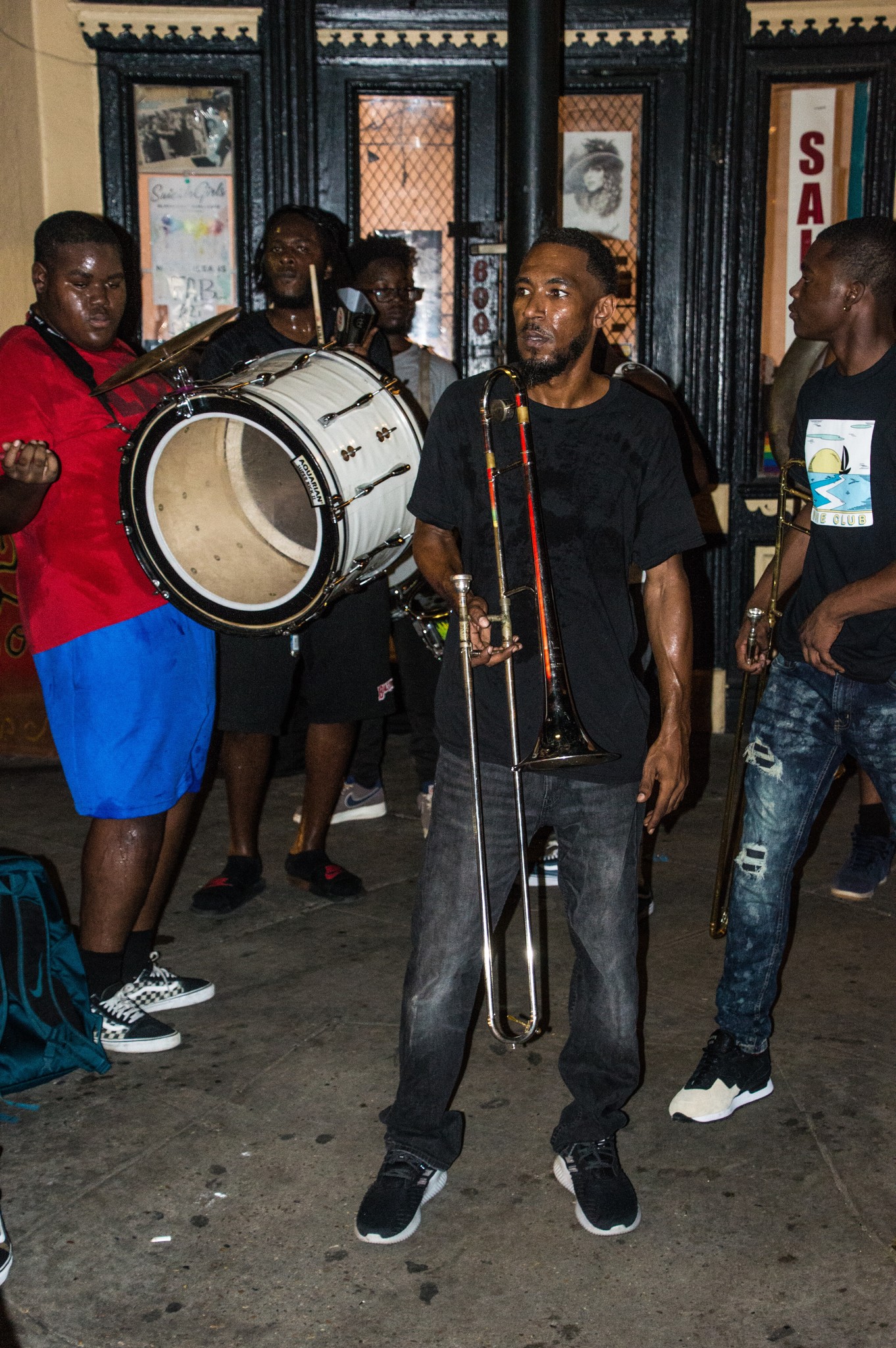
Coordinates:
[360,287,425,303]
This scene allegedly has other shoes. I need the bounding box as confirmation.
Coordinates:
[286,862,367,900]
[189,874,268,916]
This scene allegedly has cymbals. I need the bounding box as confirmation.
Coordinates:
[86,306,246,398]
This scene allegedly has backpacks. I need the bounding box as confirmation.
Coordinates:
[0,847,112,1096]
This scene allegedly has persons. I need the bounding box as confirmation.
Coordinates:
[656,215,896,1123]
[0,212,216,1055]
[191,202,462,915]
[354,226,719,1244]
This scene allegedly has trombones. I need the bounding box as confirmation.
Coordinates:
[449,367,609,1047]
[707,458,811,941]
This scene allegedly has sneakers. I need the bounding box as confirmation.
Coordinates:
[122,960,215,1012]
[514,848,560,888]
[417,783,432,841]
[831,823,896,899]
[354,1151,448,1243]
[553,1135,641,1235]
[91,984,181,1054]
[668,1029,774,1122]
[293,782,386,825]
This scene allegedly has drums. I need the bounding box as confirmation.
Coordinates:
[116,343,434,636]
